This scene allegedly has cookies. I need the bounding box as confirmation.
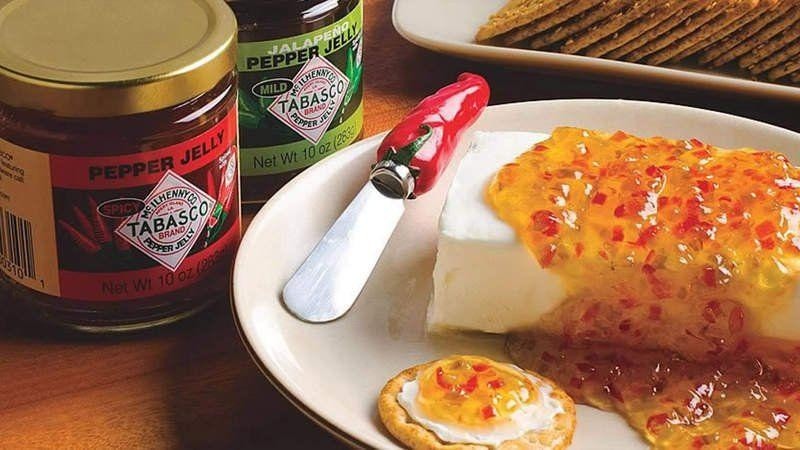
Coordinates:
[377,353,577,450]
[474,0,800,86]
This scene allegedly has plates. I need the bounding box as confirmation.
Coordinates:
[390,0,800,97]
[232,99,800,450]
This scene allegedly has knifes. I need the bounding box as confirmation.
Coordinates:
[281,72,490,322]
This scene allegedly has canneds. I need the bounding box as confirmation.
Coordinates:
[0,0,366,330]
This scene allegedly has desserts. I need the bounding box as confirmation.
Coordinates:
[421,126,800,372]
